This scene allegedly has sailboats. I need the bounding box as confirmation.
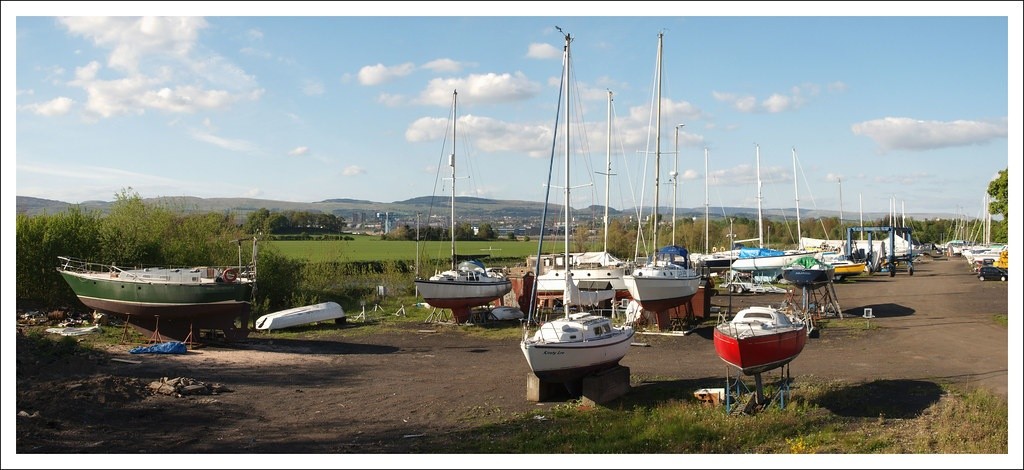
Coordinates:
[685,144,927,272]
[934,189,1008,265]
[414,93,511,309]
[519,33,635,377]
[623,33,702,310]
[507,31,627,308]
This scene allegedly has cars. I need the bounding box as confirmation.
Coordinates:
[977,267,1008,282]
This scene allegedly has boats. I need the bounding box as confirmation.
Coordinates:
[255,301,344,333]
[781,266,835,291]
[713,307,806,371]
[55,253,255,321]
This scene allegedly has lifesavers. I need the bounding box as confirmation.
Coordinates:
[224,268,238,283]
